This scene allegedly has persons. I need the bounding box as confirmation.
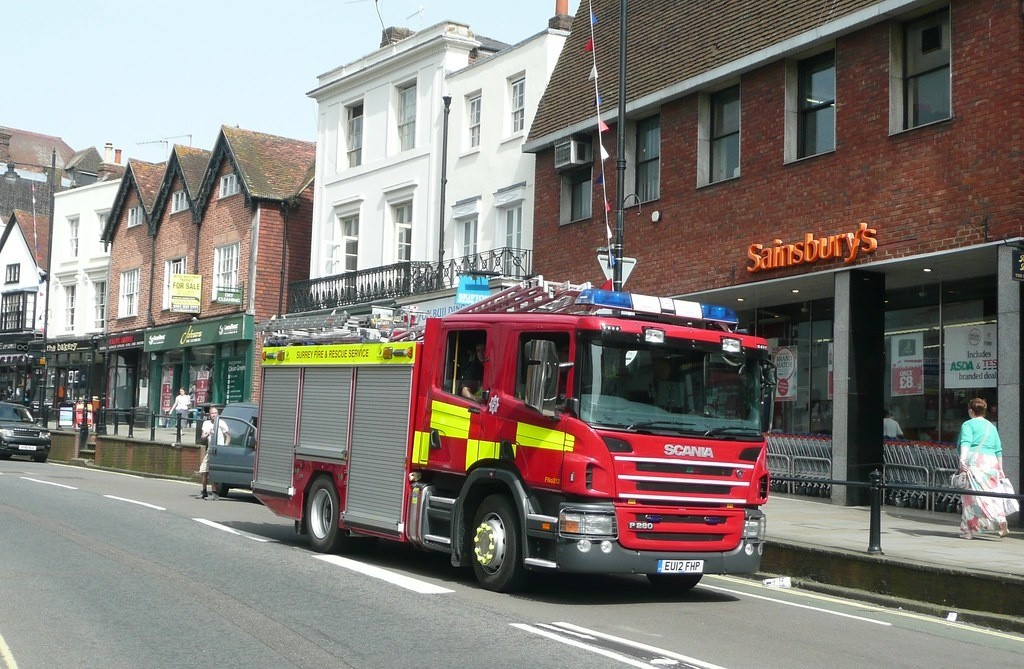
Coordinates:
[459,341,487,401]
[882,410,904,440]
[169,388,191,435]
[637,354,688,414]
[957,398,1010,539]
[194,406,231,500]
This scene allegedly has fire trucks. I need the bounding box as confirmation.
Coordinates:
[250,274,778,594]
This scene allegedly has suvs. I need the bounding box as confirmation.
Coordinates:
[0,399,52,463]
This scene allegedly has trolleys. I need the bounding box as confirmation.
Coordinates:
[763,430,965,515]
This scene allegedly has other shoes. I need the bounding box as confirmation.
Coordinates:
[195,492,208,499]
[181,433,183,436]
[999,520,1009,536]
[959,533,972,540]
[204,493,219,501]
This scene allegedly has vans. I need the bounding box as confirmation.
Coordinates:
[208,400,260,497]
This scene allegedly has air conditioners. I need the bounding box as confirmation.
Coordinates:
[553,140,591,170]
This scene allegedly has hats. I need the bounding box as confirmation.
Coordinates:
[472,335,485,351]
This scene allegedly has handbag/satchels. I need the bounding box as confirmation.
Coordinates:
[998,477,1019,516]
[948,472,966,489]
[165,413,173,428]
